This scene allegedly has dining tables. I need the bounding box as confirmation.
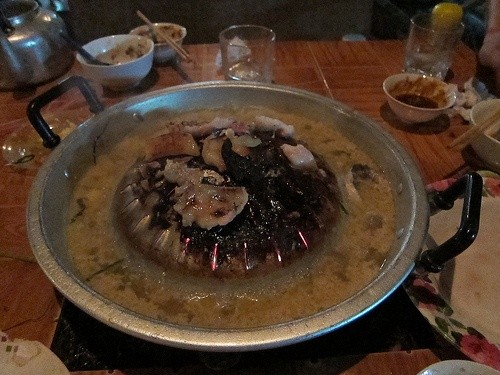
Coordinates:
[1,40,499,375]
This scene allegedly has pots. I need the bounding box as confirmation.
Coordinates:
[25,74,482,353]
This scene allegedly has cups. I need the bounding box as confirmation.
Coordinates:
[402,14,466,80]
[219,25,275,85]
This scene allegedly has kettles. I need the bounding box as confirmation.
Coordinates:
[0,0,73,89]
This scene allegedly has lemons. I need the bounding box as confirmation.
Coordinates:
[430,3,463,29]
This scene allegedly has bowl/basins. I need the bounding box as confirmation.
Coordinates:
[381,73,457,125]
[469,99,499,169]
[129,22,188,61]
[76,33,154,92]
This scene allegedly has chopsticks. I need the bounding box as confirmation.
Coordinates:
[137,10,193,63]
[446,108,500,152]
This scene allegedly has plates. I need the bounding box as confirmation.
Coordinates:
[402,177,500,370]
[416,360,500,375]
[0,332,70,375]
